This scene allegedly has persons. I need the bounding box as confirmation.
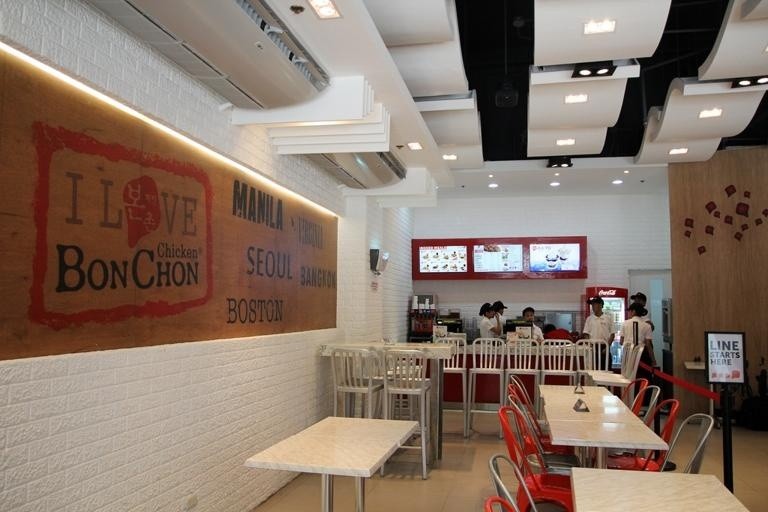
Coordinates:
[543,322,569,339]
[581,295,619,352]
[479,300,503,345]
[491,300,507,336]
[629,291,655,349]
[619,302,657,366]
[521,305,544,345]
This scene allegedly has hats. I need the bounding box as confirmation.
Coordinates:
[478,303,492,316]
[522,307,534,316]
[492,301,507,310]
[630,292,646,302]
[624,302,642,311]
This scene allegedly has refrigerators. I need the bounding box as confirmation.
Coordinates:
[585,287,628,373]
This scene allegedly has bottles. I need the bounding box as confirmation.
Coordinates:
[610,343,622,364]
[603,300,620,331]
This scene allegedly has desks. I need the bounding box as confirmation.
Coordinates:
[569,463,751,512]
[244,416,420,511]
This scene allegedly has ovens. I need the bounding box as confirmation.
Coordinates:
[661,298,672,338]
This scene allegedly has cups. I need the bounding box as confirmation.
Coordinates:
[411,295,435,309]
[545,254,559,268]
[558,254,568,264]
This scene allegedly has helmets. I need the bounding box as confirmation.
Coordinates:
[586,297,603,305]
